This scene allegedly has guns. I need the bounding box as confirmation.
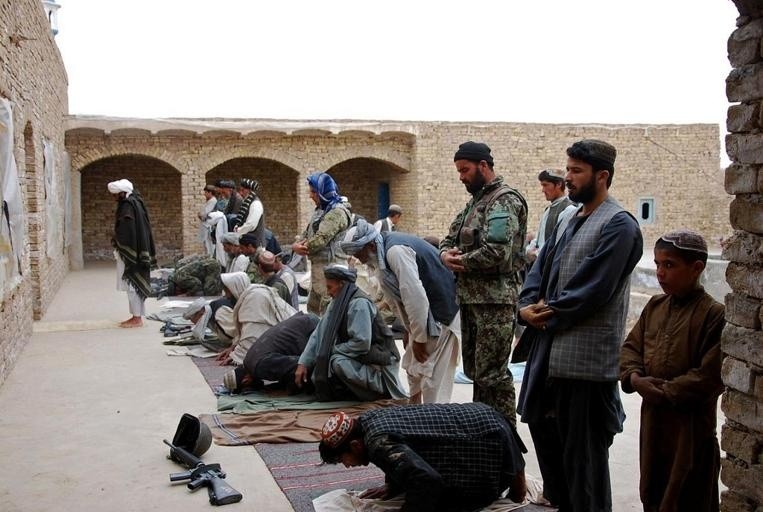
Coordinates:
[162,439,243,508]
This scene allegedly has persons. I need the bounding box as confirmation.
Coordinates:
[207,179,244,275]
[254,251,293,307]
[206,211,284,273]
[337,196,364,228]
[372,204,402,233]
[197,185,218,258]
[223,311,320,396]
[214,271,298,366]
[221,232,251,273]
[438,141,529,429]
[618,230,726,511]
[510,140,644,512]
[182,295,237,347]
[293,262,410,401]
[291,172,354,318]
[524,167,573,264]
[318,405,527,512]
[214,179,227,212]
[108,179,157,328]
[167,257,227,297]
[234,178,266,251]
[239,233,276,288]
[274,257,300,312]
[339,219,463,404]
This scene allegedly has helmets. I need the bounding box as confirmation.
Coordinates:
[170,413,213,462]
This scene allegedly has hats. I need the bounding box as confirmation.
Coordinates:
[240,178,259,191]
[218,180,236,188]
[653,228,708,254]
[454,141,494,164]
[389,204,401,213]
[239,234,257,244]
[183,298,212,340]
[107,179,133,198]
[223,370,237,390]
[547,168,565,179]
[204,185,216,191]
[220,232,239,244]
[259,251,275,264]
[340,219,387,271]
[575,139,618,164]
[322,263,358,283]
[321,411,353,448]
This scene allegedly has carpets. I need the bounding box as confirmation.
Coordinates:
[168,294,557,512]
